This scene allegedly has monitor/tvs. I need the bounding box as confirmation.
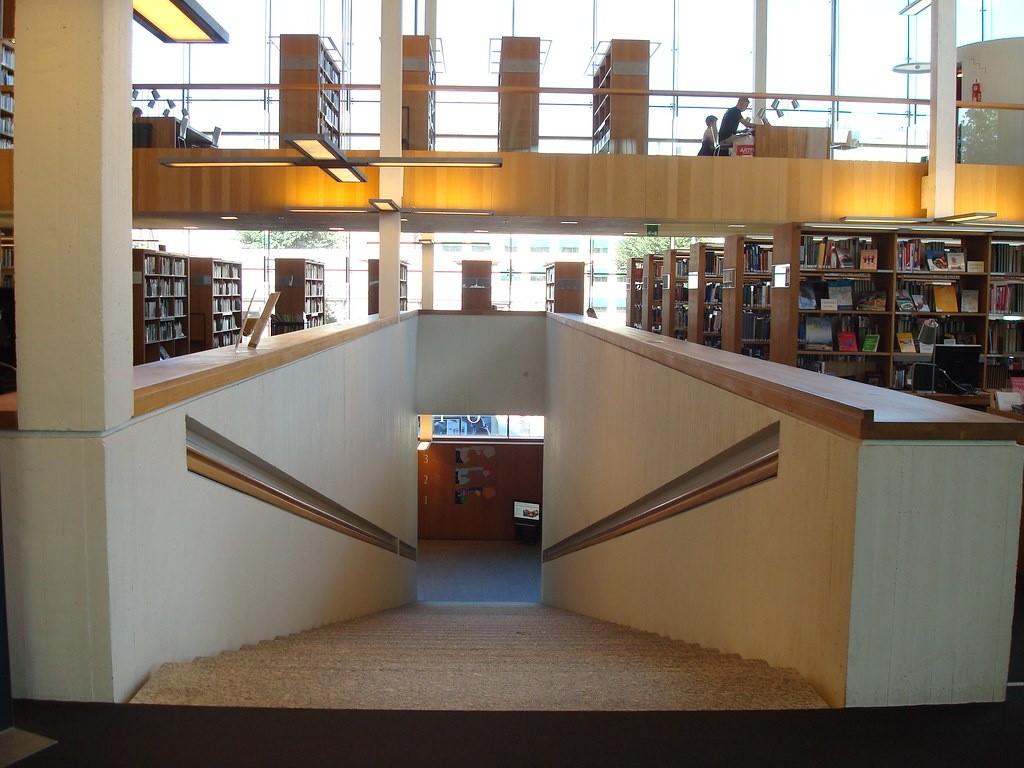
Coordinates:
[934,345,981,394]
[513,500,542,525]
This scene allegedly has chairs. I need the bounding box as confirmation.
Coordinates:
[830,129,860,151]
[710,122,733,156]
[176,117,190,148]
[202,126,223,148]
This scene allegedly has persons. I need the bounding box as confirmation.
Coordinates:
[586,308,597,318]
[697,115,719,156]
[719,96,755,156]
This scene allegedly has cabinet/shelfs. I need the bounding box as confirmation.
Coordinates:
[641,253,663,332]
[379,34,437,151]
[592,38,651,155]
[274,258,326,334]
[769,221,1024,405]
[545,262,584,315]
[720,234,774,354]
[0,37,15,142]
[461,260,492,310]
[750,125,831,159]
[279,33,342,149]
[687,242,725,345]
[132,249,191,366]
[662,249,688,337]
[626,258,644,327]
[189,256,242,353]
[497,35,541,152]
[368,259,408,315]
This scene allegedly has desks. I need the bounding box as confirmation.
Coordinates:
[903,388,1012,404]
[133,117,213,148]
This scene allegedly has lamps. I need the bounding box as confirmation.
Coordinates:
[132,90,139,100]
[162,109,170,117]
[283,132,368,183]
[147,100,156,109]
[368,156,504,169]
[152,90,161,100]
[756,108,766,119]
[937,213,997,222]
[790,100,799,109]
[167,100,176,109]
[746,117,752,122]
[776,108,785,118]
[917,317,940,393]
[159,157,298,168]
[368,198,401,212]
[133,0,229,44]
[770,100,781,110]
[182,109,189,116]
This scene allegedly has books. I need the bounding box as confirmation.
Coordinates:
[318,46,339,147]
[400,267,407,311]
[546,267,554,312]
[213,265,242,348]
[0,46,15,149]
[795,234,1024,413]
[634,262,643,323]
[305,264,324,329]
[703,245,773,360]
[652,258,688,340]
[144,255,188,343]
